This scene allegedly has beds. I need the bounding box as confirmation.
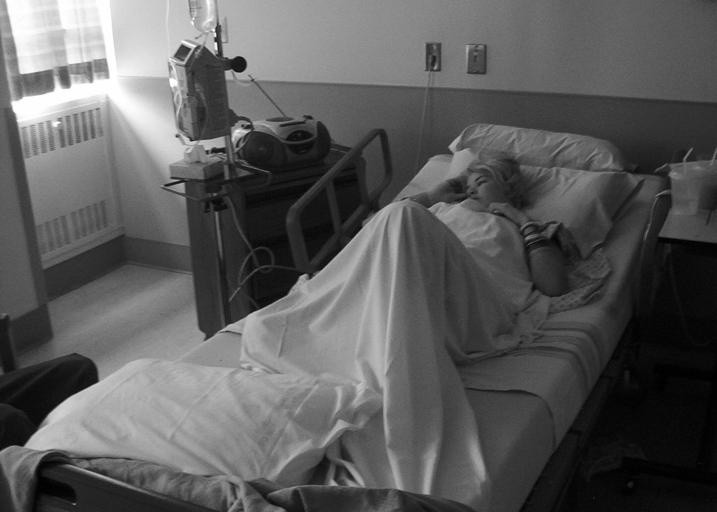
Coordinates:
[0,127,671,511]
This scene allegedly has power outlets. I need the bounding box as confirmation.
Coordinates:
[424,42,441,72]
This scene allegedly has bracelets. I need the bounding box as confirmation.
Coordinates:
[519,221,545,250]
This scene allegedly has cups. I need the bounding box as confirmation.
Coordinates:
[669,164,708,216]
[700,161,717,211]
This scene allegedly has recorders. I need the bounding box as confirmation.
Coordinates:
[231,114,332,172]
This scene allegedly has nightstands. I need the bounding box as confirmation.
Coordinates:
[619,202,717,486]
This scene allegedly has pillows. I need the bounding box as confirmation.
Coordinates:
[447,123,628,172]
[444,148,644,259]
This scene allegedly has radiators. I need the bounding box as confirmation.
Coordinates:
[15,93,125,270]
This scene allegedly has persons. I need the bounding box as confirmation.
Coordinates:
[367,154,569,368]
